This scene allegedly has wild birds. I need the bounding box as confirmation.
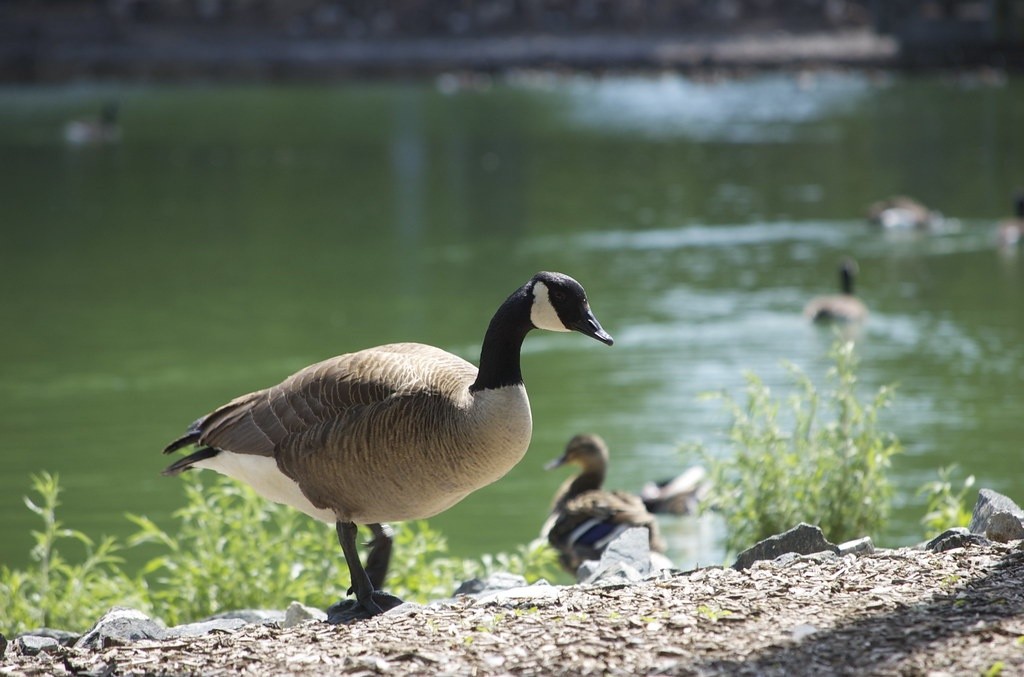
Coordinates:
[993,198,1024,254]
[800,259,870,329]
[641,466,713,518]
[540,432,666,569]
[868,195,944,233]
[159,268,617,631]
